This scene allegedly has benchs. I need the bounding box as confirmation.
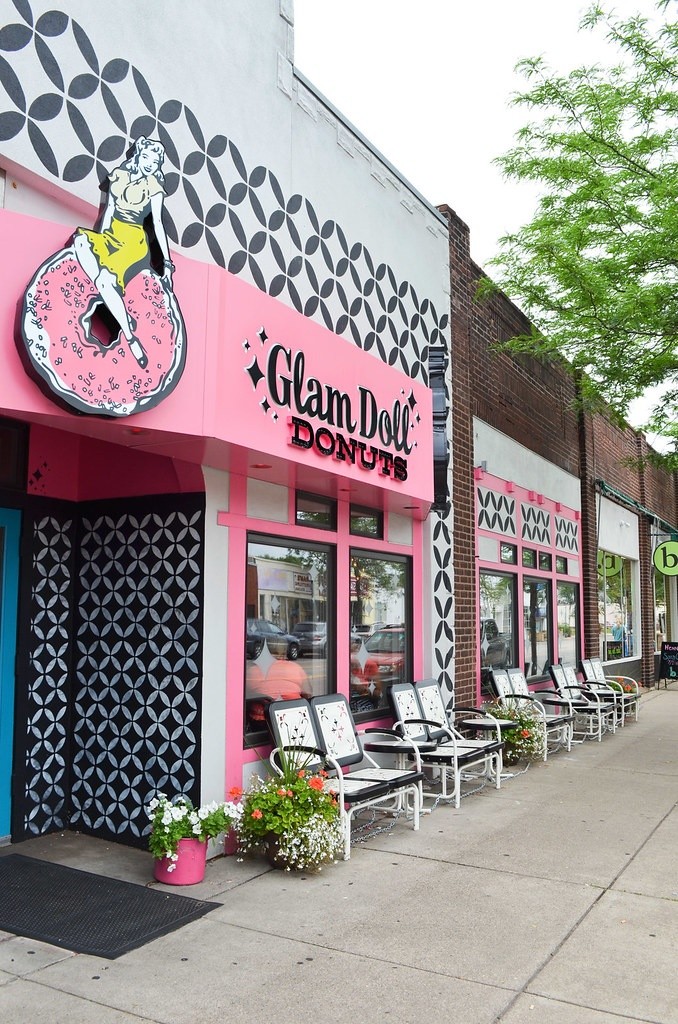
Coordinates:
[262,693,425,863]
[386,678,505,809]
[550,663,617,742]
[490,667,576,763]
[578,657,642,727]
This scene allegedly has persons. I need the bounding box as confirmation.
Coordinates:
[611,614,626,641]
[349,639,383,699]
[246,638,312,732]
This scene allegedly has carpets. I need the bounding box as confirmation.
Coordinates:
[0,853,222,960]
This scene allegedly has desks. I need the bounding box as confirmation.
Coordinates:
[542,697,589,747]
[581,689,622,732]
[364,740,431,816]
[459,718,518,783]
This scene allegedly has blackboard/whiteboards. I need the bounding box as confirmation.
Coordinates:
[603,641,622,661]
[658,641,678,680]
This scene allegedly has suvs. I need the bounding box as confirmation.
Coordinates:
[352,621,404,643]
[246,619,301,661]
[350,623,406,695]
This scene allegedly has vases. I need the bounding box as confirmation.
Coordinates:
[491,742,522,766]
[265,830,299,871]
[154,837,207,885]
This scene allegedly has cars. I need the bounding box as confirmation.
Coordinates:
[292,621,363,659]
[480,617,512,668]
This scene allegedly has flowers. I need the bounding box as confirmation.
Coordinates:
[226,724,350,873]
[475,680,550,761]
[148,793,244,873]
